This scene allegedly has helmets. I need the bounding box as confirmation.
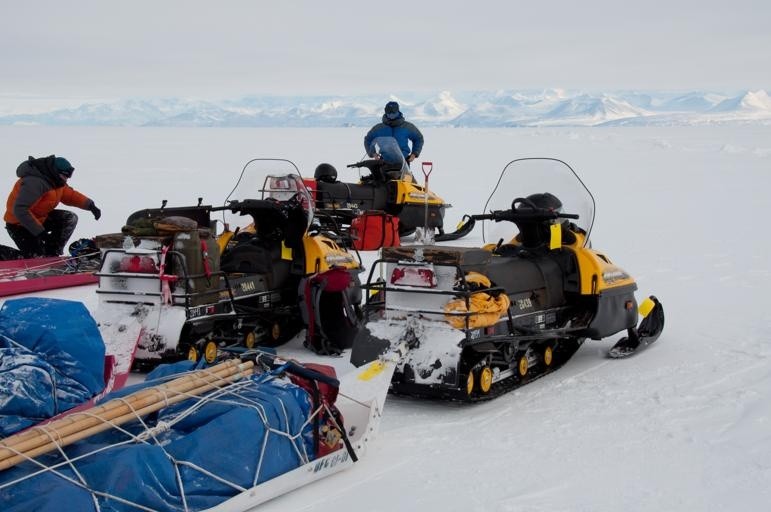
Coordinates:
[519,192,571,235]
[451,271,492,288]
[69,238,99,261]
[314,164,337,182]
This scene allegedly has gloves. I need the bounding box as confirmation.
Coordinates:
[89,203,101,220]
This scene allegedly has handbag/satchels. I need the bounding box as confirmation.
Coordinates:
[220,227,294,289]
[350,209,400,250]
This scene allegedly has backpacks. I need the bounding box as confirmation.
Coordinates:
[298,266,359,355]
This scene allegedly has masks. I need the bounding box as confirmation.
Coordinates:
[387,112,399,120]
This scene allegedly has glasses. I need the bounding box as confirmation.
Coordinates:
[385,107,399,113]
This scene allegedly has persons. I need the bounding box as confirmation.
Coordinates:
[1,155,102,261]
[364,102,426,173]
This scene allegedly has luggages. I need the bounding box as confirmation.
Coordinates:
[170,227,221,307]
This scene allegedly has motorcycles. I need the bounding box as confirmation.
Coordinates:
[339,158,665,408]
[90,136,453,356]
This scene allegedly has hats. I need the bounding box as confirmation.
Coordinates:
[385,102,399,108]
[54,157,73,177]
